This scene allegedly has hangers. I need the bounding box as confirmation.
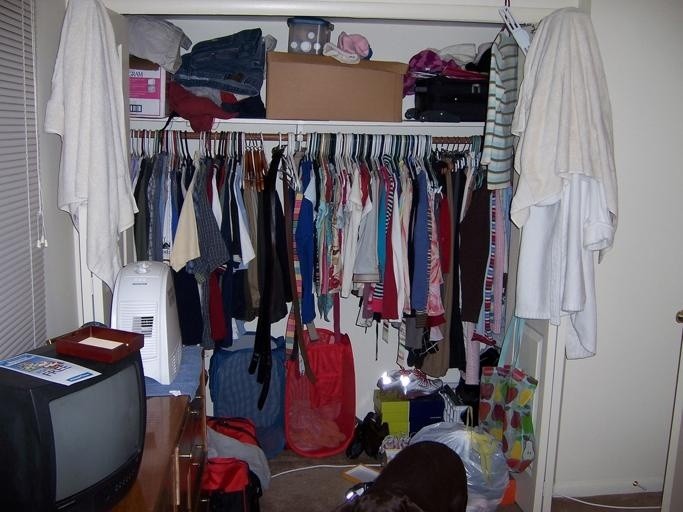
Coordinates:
[130,129,486,190]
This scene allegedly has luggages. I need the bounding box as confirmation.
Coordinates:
[403,76,488,123]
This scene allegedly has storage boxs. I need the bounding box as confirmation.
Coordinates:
[128,64,173,118]
[371,390,444,443]
[265,18,410,121]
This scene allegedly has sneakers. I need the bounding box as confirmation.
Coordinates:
[378,368,443,396]
[346,412,388,459]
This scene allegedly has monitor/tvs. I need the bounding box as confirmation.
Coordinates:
[0,341,147,512]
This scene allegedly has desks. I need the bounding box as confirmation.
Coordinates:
[105,351,208,512]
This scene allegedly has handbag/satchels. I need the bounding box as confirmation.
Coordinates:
[197,414,261,512]
[480,367,538,473]
[441,384,469,425]
